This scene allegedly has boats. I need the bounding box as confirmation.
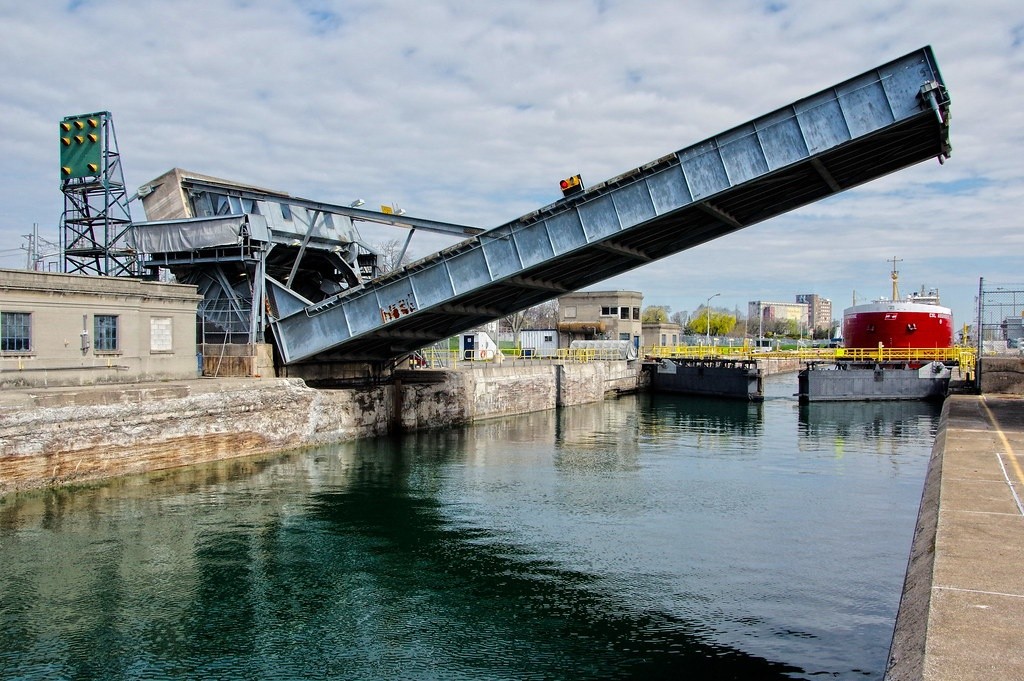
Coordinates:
[840,254,955,369]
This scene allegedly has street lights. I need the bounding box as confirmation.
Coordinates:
[997,287,1015,315]
[801,313,811,348]
[988,300,1002,322]
[707,293,720,345]
[759,303,773,353]
